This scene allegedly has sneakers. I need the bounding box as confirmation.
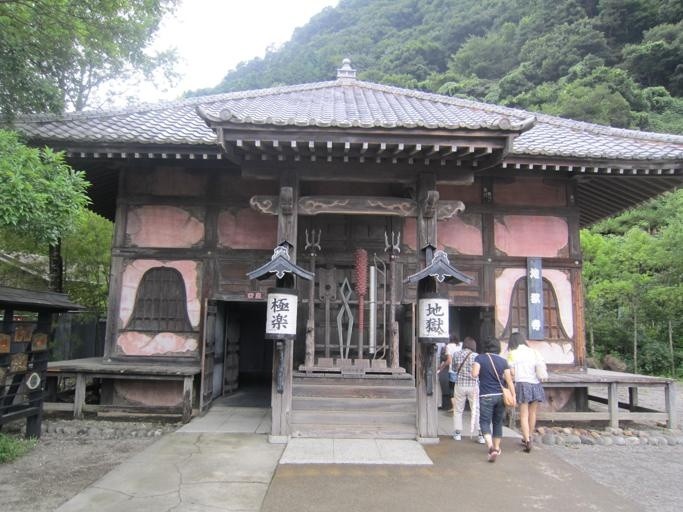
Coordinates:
[488,448,501,462]
[521,440,531,451]
[477,435,485,443]
[452,432,462,441]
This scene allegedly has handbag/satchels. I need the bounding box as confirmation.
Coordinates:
[503,388,513,406]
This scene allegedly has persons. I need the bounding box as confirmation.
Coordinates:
[450,335,485,444]
[506,332,547,453]
[472,335,515,463]
[435,343,451,410]
[441,336,462,383]
